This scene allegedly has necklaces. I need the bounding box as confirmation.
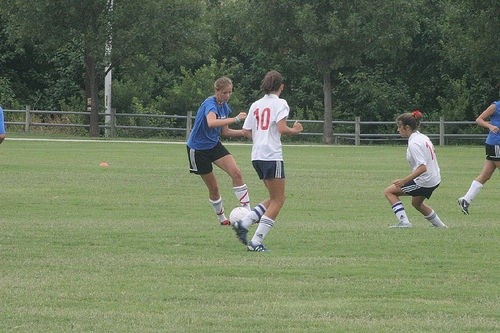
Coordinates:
[218,102,224,105]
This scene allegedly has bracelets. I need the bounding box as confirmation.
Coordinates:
[235,115,240,125]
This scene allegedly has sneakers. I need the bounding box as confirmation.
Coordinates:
[247,240,272,252]
[457,196,470,216]
[231,220,249,245]
[389,221,413,228]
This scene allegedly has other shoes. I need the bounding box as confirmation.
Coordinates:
[221,219,230,225]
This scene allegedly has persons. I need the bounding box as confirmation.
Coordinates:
[0,107,6,144]
[186,77,253,225]
[231,70,303,252]
[457,100,500,215]
[383,110,449,227]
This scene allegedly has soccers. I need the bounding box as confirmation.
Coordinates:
[228,206,253,229]
